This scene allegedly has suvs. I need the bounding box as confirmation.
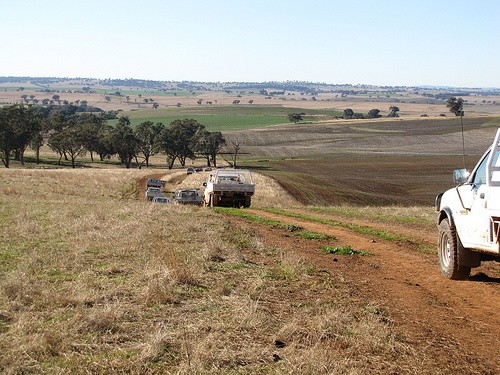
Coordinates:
[433,128,500,280]
[144,187,161,202]
[203,169,254,208]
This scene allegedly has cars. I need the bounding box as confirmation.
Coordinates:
[151,194,169,203]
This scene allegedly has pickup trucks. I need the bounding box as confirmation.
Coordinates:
[175,189,203,207]
[147,177,163,190]
